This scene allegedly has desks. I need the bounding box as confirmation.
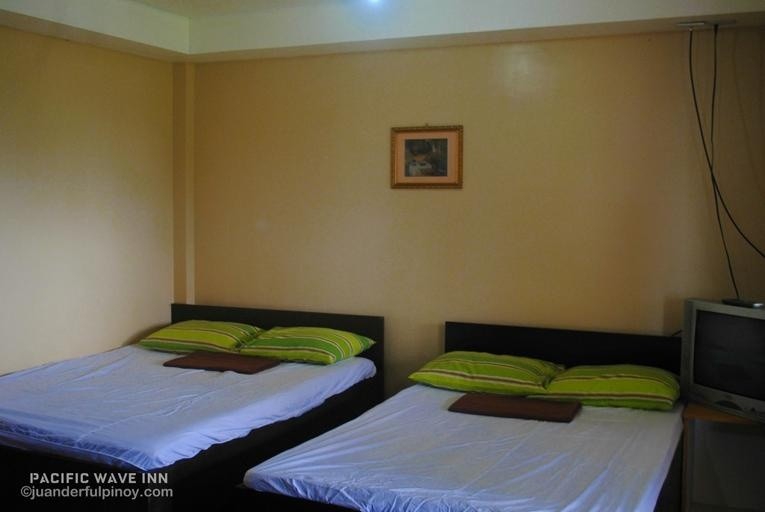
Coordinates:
[681,402,756,511]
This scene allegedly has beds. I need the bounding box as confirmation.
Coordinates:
[0,303,684,512]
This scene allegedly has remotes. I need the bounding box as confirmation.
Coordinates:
[721,298,764,308]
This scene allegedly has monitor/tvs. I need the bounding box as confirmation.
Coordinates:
[680,297,765,422]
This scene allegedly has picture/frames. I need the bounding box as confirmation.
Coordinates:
[390,125,463,189]
